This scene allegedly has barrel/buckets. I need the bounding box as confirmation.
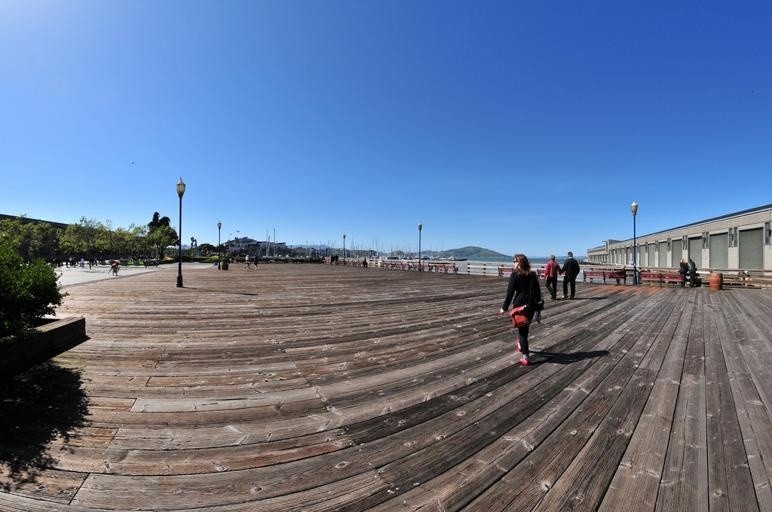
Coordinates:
[710,272,724,290]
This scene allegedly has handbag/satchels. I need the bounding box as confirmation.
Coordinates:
[508,305,529,329]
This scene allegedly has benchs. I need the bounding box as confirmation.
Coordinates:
[498,267,546,280]
[406,261,459,273]
[583,270,686,287]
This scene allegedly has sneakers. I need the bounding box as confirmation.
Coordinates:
[516,341,522,351]
[519,356,528,366]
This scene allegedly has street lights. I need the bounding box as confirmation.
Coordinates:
[631,201,639,285]
[217,220,222,270]
[175,175,186,287]
[418,224,422,271]
[343,234,346,265]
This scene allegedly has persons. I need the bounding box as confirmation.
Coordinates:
[560,252,580,300]
[112,261,119,277]
[322,255,326,265]
[43,254,98,270]
[500,254,541,365]
[244,252,250,270]
[253,253,258,270]
[544,254,561,300]
[688,259,695,287]
[678,258,688,288]
[363,259,368,268]
